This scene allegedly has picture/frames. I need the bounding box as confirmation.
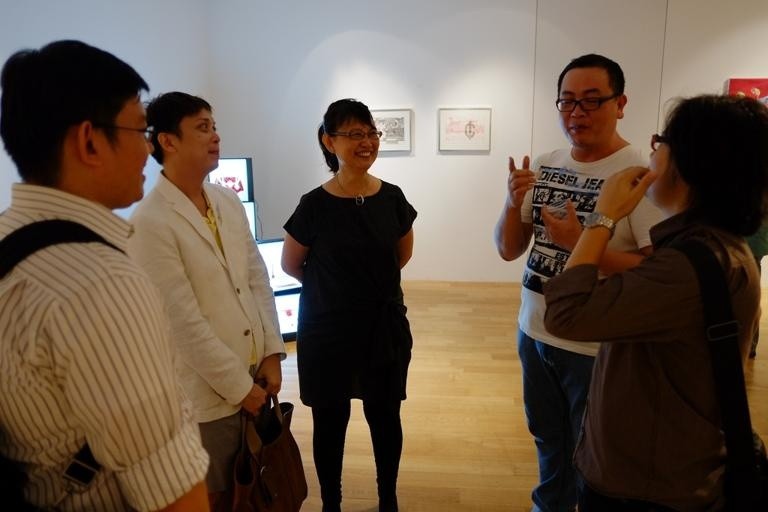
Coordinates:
[435,107,492,154]
[369,108,413,153]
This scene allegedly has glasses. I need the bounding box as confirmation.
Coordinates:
[332,129,382,140]
[95,124,154,140]
[556,92,616,112]
[651,134,673,151]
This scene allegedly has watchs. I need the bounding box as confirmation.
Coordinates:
[586,212,619,237]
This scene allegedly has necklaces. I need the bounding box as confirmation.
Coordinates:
[337,174,371,206]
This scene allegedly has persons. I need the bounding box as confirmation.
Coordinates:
[280,100,417,511]
[0,40,215,512]
[545,93,767,506]
[496,54,669,511]
[130,91,288,511]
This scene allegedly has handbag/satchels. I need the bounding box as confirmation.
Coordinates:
[232,403,307,511]
[725,430,768,512]
